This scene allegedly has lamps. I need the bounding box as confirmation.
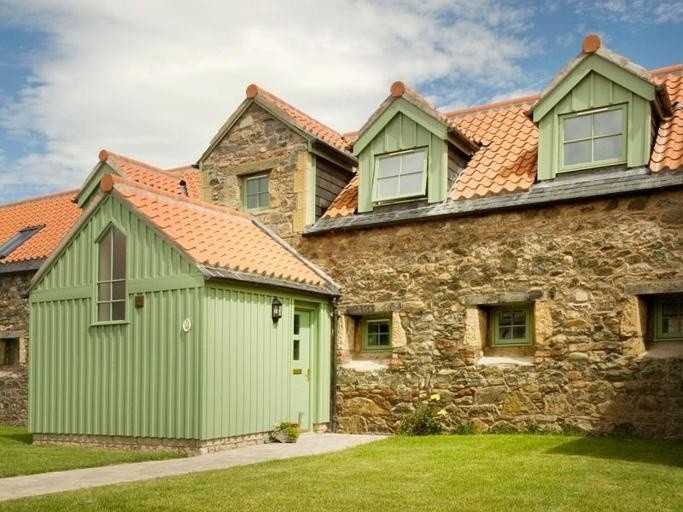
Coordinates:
[272,297,283,319]
